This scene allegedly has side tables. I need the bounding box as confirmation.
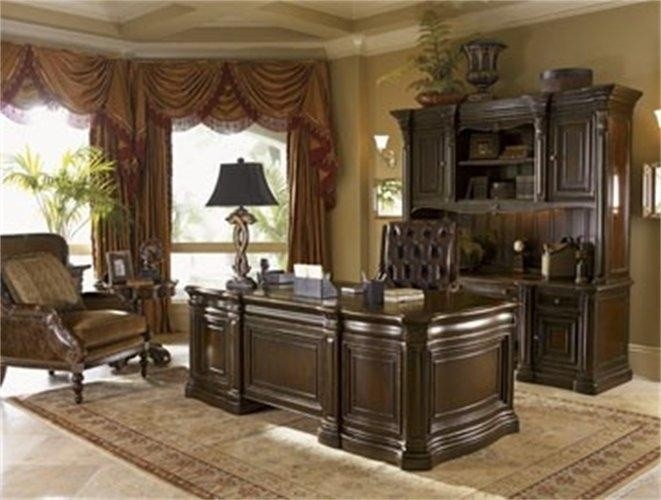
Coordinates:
[94,267,176,371]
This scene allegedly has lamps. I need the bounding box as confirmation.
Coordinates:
[373,134,395,167]
[653,104,661,132]
[204,157,279,289]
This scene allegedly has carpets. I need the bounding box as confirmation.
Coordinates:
[8,366,661,500]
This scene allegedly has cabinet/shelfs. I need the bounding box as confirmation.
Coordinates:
[388,84,644,395]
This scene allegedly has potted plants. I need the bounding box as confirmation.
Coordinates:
[4,146,142,294]
[378,11,473,104]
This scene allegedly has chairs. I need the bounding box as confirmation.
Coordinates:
[375,218,462,292]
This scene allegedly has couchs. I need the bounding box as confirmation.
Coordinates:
[0,234,147,405]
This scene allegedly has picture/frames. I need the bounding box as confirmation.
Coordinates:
[639,158,661,221]
[107,251,134,286]
[376,178,403,219]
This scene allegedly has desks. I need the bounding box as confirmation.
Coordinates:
[184,260,524,472]
